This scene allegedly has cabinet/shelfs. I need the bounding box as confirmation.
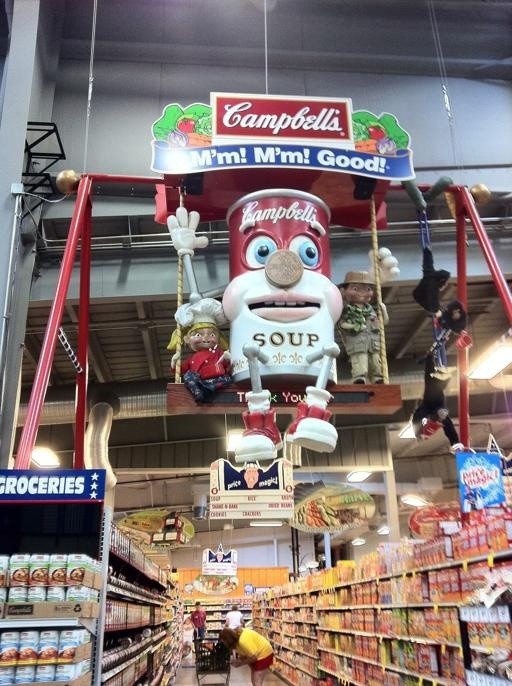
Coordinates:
[252,547,512,686]
[0,505,184,686]
[184,604,252,640]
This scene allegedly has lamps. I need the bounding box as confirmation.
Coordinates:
[399,424,416,438]
[463,325,512,380]
[377,526,389,535]
[347,471,372,482]
[30,446,61,469]
[248,520,283,527]
[352,538,365,546]
[226,428,244,451]
[401,494,427,507]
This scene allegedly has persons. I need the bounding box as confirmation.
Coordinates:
[191,601,207,642]
[170,297,232,404]
[225,606,246,659]
[218,627,274,686]
[338,269,391,385]
